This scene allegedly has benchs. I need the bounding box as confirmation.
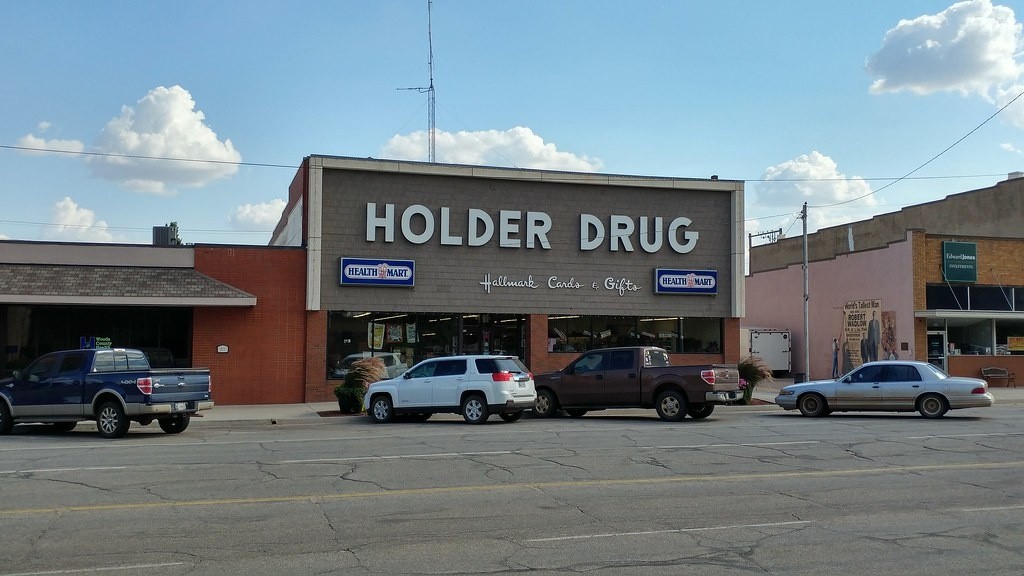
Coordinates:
[980,367,1016,388]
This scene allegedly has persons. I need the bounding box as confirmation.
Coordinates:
[833,337,840,379]
[861,311,880,364]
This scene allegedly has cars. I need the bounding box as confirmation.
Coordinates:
[774,360,995,420]
[333,352,408,381]
[364,355,538,425]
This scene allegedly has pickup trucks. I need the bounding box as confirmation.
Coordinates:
[0,348,214,439]
[532,346,744,422]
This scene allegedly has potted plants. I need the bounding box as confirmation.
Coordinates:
[334,357,389,414]
